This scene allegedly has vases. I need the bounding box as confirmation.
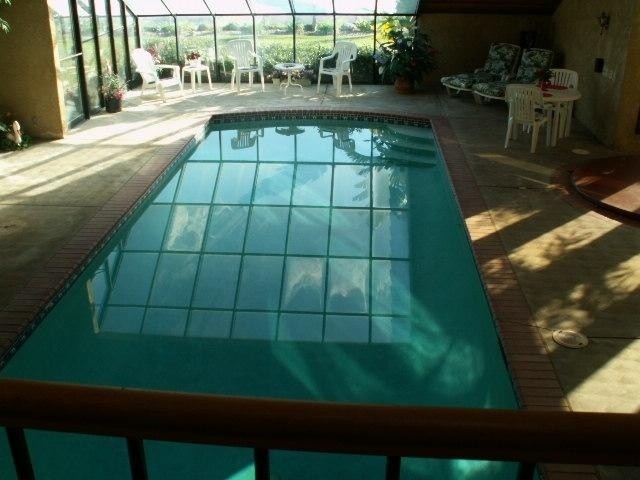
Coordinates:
[105,98,124,113]
[190,59,202,68]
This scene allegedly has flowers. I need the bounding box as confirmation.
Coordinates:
[187,51,203,62]
[100,71,131,98]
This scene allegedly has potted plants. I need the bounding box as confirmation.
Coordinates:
[375,16,446,94]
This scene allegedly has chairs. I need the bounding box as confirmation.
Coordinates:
[315,40,360,95]
[130,47,183,103]
[503,84,550,154]
[473,48,555,105]
[224,39,266,94]
[527,69,579,139]
[440,42,520,103]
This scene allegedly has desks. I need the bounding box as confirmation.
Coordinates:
[181,64,214,94]
[531,84,582,147]
[274,63,306,94]
